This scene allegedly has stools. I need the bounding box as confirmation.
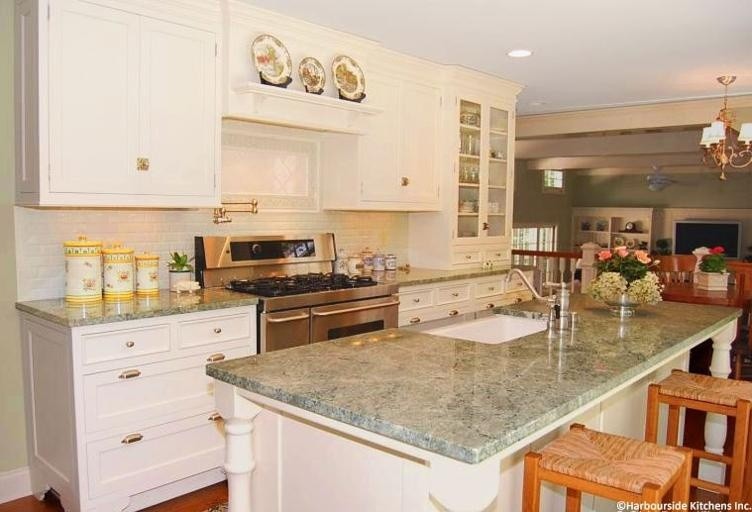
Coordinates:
[520,424,695,511]
[644,362,752,510]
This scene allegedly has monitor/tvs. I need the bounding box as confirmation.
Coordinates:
[672,221,742,260]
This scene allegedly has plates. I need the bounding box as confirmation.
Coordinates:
[250,32,291,87]
[458,201,504,215]
[256,295,399,353]
[297,58,328,96]
[330,54,365,102]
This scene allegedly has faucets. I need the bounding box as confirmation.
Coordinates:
[505,268,571,330]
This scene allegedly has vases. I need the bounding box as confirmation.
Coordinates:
[696,269,729,293]
[601,289,641,319]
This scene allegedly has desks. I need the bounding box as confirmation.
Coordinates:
[661,278,751,378]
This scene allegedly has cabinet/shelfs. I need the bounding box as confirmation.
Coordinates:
[567,201,656,291]
[404,61,526,270]
[17,297,259,512]
[474,266,539,312]
[399,272,475,329]
[12,1,230,211]
[653,249,752,287]
[316,32,450,215]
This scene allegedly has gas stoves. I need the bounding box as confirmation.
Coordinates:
[224,275,403,312]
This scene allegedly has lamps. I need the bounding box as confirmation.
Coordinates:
[696,72,752,182]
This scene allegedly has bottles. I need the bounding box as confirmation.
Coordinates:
[550,282,581,329]
[546,330,577,372]
[459,161,478,185]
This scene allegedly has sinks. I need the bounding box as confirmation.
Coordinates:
[419,313,548,345]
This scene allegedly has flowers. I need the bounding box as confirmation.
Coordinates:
[587,244,663,307]
[700,246,727,274]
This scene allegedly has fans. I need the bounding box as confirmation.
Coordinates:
[635,166,704,193]
